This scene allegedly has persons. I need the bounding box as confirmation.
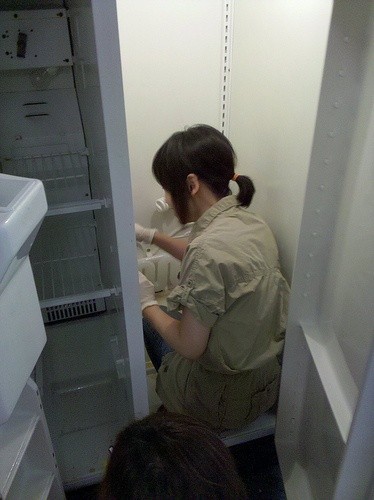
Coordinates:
[134,122,290,434]
[98,412,248,500]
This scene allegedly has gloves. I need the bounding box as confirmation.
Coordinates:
[138,271,159,314]
[134,222,157,244]
